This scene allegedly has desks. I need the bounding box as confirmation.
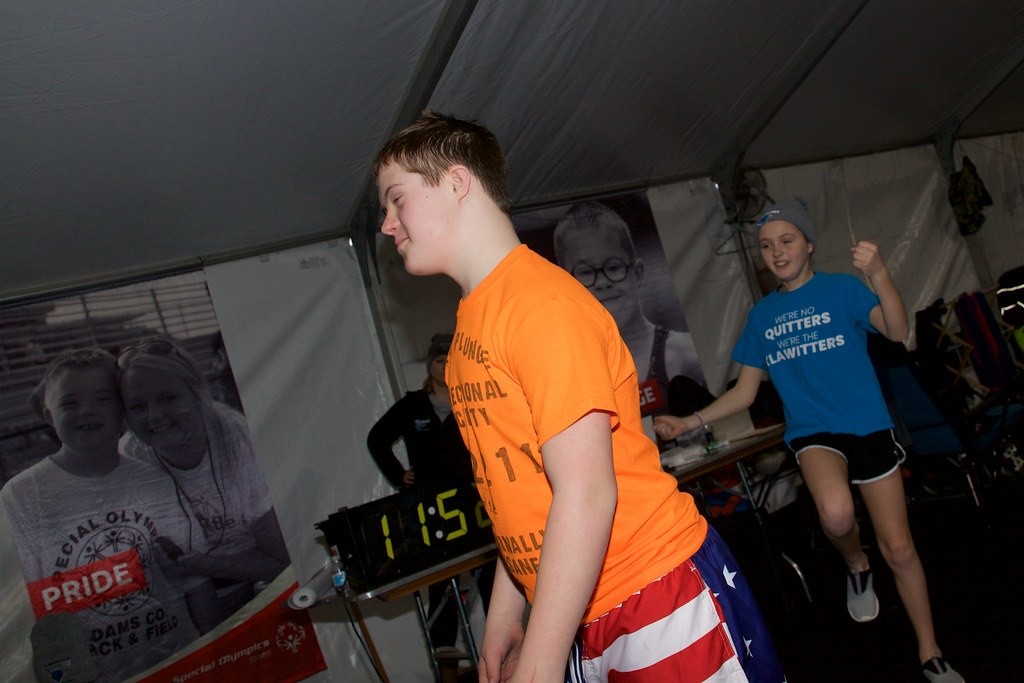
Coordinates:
[354,425,859,683]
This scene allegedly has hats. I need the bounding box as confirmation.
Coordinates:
[425,334,451,369]
[755,199,816,255]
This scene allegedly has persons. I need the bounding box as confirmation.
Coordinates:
[553,200,711,453]
[111,335,295,637]
[370,109,787,681]
[367,332,497,683]
[1,348,199,681]
[652,197,968,682]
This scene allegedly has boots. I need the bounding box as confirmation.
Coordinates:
[434,644,463,683]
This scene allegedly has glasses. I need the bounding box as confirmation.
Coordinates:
[572,255,636,287]
[115,341,197,377]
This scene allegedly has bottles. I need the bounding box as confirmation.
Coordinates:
[330,556,350,596]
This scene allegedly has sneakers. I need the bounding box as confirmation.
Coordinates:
[919,655,966,683]
[846,562,879,625]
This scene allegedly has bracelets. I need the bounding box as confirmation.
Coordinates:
[694,410,709,430]
